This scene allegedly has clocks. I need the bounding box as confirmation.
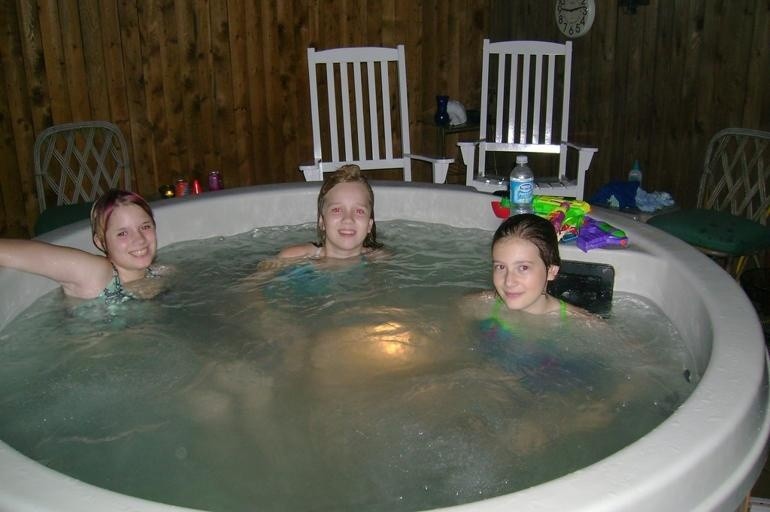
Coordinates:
[554,0,596,40]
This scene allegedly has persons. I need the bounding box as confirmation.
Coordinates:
[273,163,396,268]
[0,187,278,441]
[456,210,640,459]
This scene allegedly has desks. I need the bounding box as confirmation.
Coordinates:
[594,199,683,223]
[417,108,497,184]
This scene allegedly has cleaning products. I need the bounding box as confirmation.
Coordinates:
[629,159,641,181]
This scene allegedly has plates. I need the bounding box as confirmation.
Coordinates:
[158,184,176,198]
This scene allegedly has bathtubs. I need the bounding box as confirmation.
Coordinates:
[0,181,767,511]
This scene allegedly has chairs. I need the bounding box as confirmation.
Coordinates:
[33,120,133,237]
[457,39,598,201]
[646,128,770,284]
[300,44,454,185]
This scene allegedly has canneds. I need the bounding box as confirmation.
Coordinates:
[175,176,189,197]
[207,169,224,191]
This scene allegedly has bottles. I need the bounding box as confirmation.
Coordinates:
[434,94,450,126]
[509,154,534,217]
[628,160,641,188]
